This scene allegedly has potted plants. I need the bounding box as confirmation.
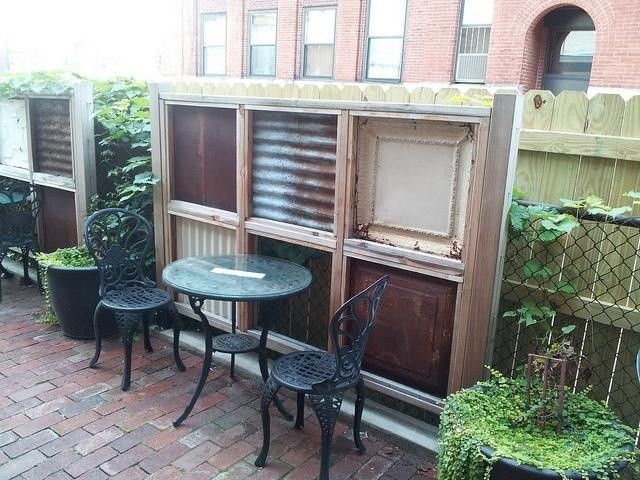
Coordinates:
[431,363,640,480]
[33,245,142,345]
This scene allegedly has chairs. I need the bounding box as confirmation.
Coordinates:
[254,273,390,480]
[0,182,44,303]
[83,207,189,391]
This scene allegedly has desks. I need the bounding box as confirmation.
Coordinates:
[163,254,313,427]
[0,191,34,280]
